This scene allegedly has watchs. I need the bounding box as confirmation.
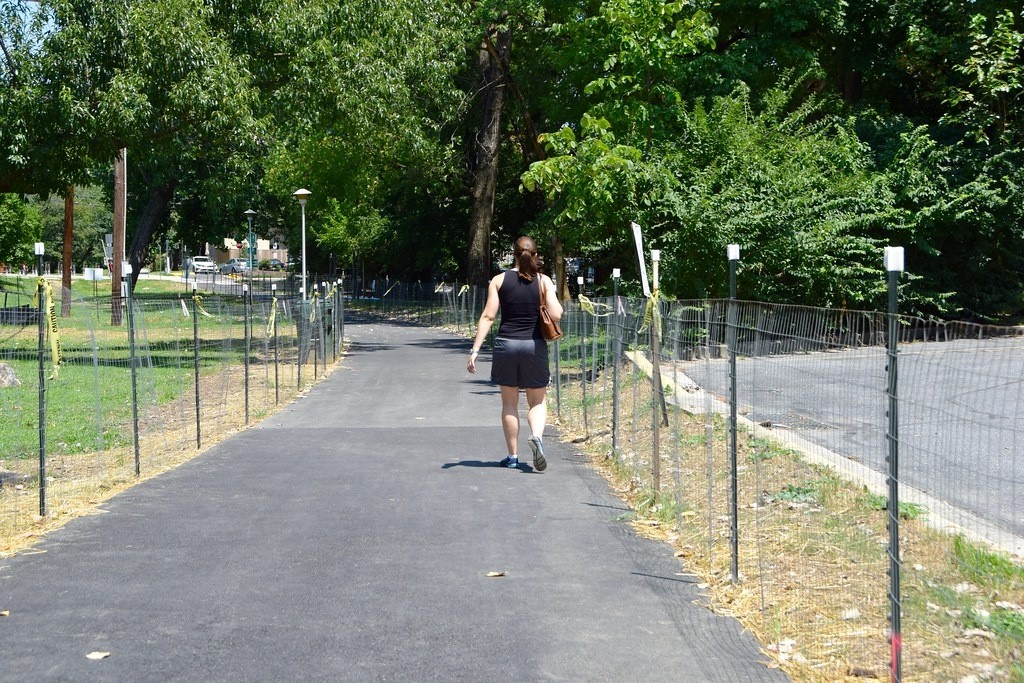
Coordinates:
[469,349,479,355]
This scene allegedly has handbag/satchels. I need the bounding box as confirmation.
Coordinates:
[539,273,563,342]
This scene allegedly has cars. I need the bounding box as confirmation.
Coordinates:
[259,259,287,271]
[220,259,249,275]
[190,256,218,274]
[562,257,593,294]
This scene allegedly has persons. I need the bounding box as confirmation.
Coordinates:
[466,236,563,471]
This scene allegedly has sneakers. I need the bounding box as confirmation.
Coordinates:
[500,457,519,468]
[528,435,547,471]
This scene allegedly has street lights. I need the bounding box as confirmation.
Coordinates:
[293,188,313,300]
[244,208,258,304]
